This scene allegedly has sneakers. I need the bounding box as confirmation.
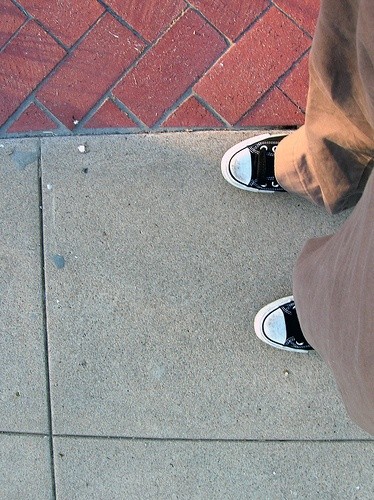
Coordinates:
[220,134,289,193]
[252,294,316,354]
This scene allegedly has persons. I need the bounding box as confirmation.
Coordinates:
[220,0,374,435]
